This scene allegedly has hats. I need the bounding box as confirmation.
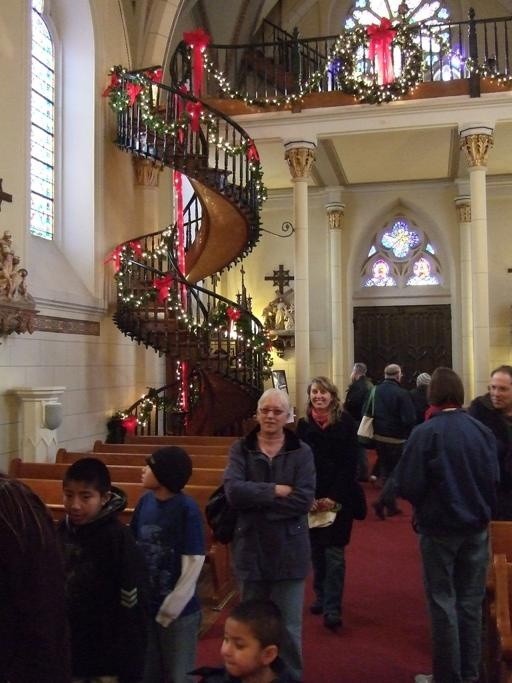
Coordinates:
[417,372,432,383]
[145,445,193,493]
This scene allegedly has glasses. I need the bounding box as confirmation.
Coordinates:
[260,407,284,415]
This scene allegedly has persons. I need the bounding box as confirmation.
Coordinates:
[467,365,512,523]
[407,372,433,429]
[295,376,366,628]
[196,596,297,683]
[343,363,374,463]
[127,446,206,682]
[52,456,148,683]
[1,472,86,683]
[223,387,317,683]
[374,367,500,680]
[366,364,416,521]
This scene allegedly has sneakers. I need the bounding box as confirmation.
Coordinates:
[414,672,437,682]
[388,507,403,518]
[325,613,342,626]
[370,498,382,521]
[310,602,322,614]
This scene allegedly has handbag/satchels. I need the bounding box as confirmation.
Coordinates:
[205,484,235,545]
[309,503,338,531]
[356,415,376,442]
